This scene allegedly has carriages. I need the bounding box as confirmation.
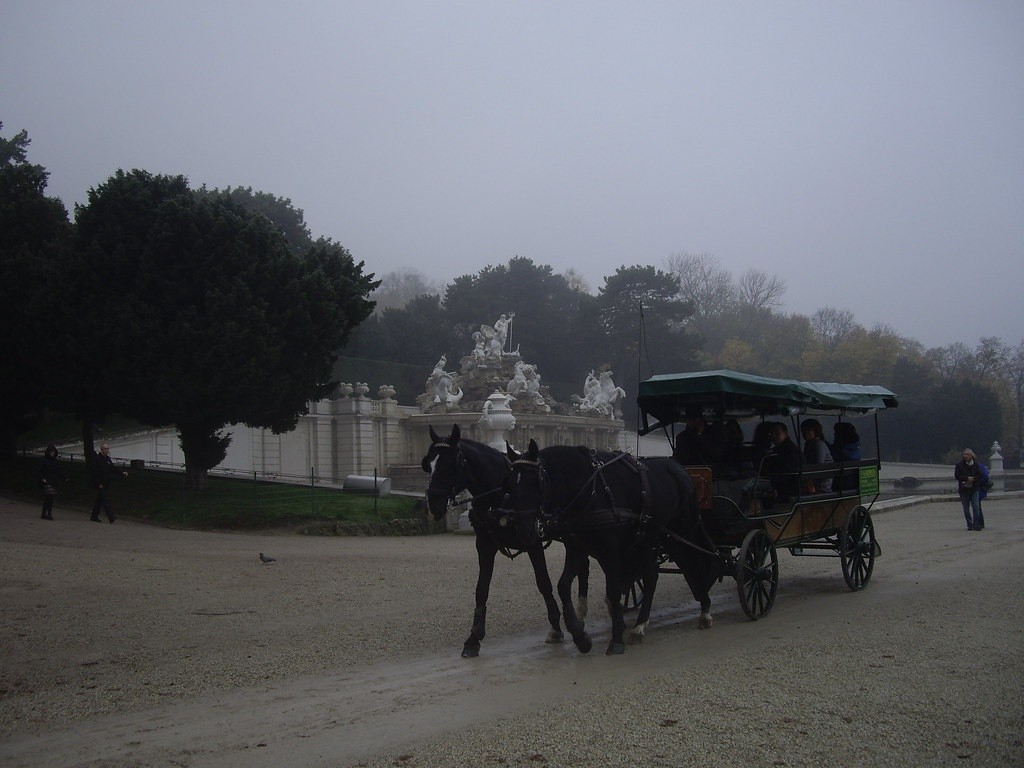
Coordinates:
[421,368,897,658]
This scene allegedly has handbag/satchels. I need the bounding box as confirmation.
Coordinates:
[801,480,816,494]
[982,480,993,491]
[961,482,973,488]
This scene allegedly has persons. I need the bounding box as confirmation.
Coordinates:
[955,448,993,531]
[90,443,128,524]
[676,404,861,497]
[39,445,69,520]
[494,314,513,353]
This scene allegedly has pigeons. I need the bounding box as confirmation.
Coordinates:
[260,553,276,564]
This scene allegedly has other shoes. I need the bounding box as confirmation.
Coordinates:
[975,527,981,531]
[90,517,101,522]
[48,517,53,520]
[41,515,47,519]
[968,527,974,530]
[110,516,117,523]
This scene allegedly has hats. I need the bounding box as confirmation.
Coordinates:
[681,407,702,417]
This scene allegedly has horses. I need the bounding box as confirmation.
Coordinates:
[505,438,711,657]
[421,424,624,659]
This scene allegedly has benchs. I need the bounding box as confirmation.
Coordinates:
[762,458,877,507]
